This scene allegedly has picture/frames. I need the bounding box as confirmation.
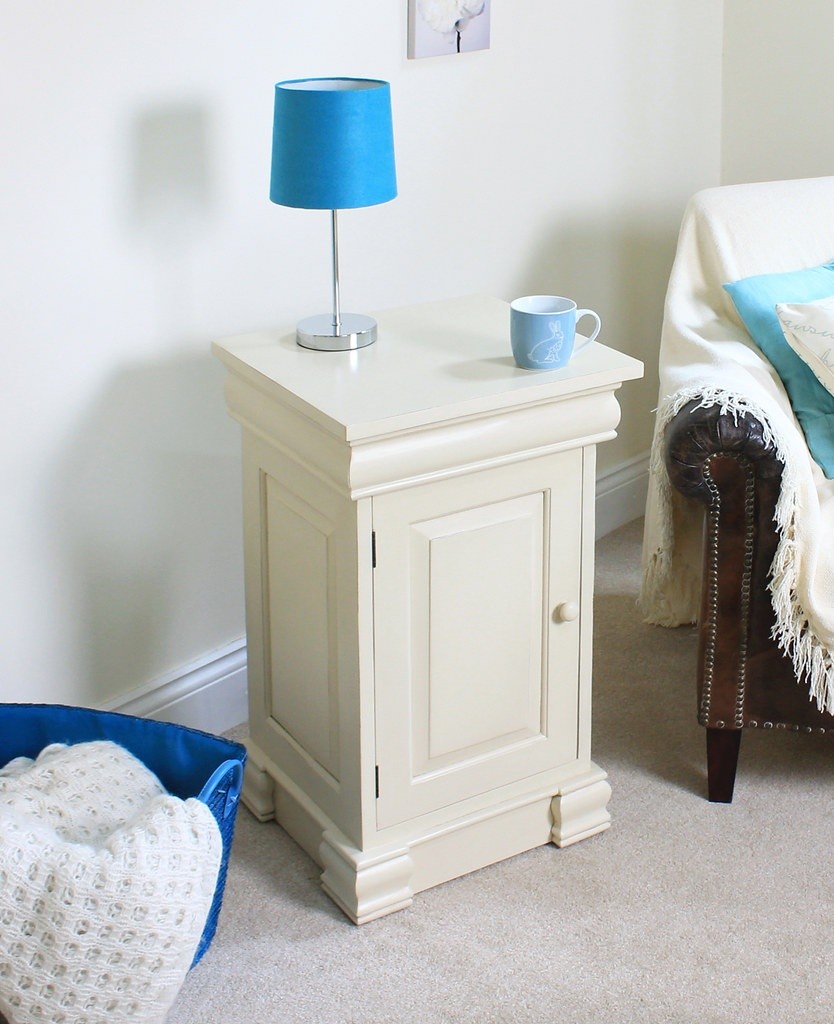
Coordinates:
[408,0,491,60]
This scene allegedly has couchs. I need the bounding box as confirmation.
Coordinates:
[643,175,833,804]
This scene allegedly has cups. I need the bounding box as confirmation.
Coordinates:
[510,295,601,372]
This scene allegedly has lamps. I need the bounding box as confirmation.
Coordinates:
[269,76,399,352]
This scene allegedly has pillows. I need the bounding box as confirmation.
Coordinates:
[777,294,833,399]
[723,260,833,483]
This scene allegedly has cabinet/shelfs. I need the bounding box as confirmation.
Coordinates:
[211,296,645,925]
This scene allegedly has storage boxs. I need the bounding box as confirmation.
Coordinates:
[1,701,248,973]
